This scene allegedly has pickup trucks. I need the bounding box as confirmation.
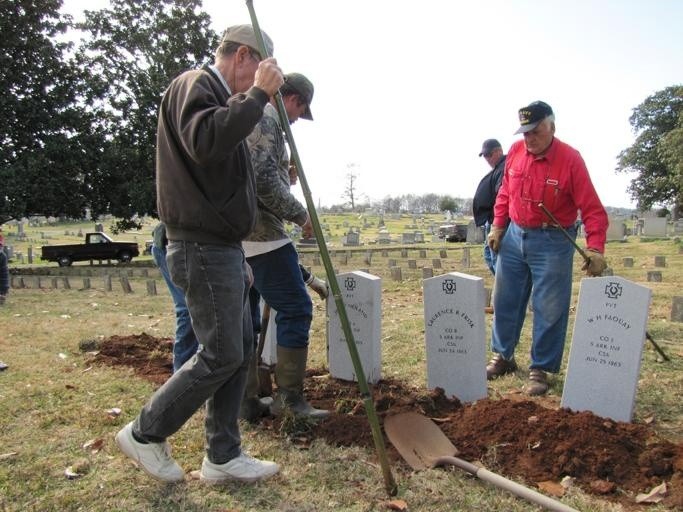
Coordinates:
[40,232,139,267]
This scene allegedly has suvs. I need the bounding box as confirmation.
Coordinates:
[440,224,467,243]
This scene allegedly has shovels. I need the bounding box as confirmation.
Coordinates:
[383,412,581,512]
[255,302,272,398]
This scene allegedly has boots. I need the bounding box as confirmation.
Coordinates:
[238,347,270,423]
[270,344,331,422]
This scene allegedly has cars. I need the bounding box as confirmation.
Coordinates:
[145,240,153,254]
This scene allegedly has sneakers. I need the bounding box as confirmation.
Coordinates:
[200,450,279,483]
[115,419,186,484]
[526,368,547,394]
[486,352,516,379]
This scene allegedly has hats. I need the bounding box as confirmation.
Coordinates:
[222,24,287,82]
[513,100,552,136]
[284,73,314,120]
[479,139,501,156]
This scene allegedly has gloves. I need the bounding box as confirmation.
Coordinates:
[488,224,506,251]
[306,276,329,300]
[581,248,608,277]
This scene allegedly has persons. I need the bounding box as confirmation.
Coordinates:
[471,137,508,277]
[111,22,328,485]
[486,101,610,397]
[0,234,11,370]
